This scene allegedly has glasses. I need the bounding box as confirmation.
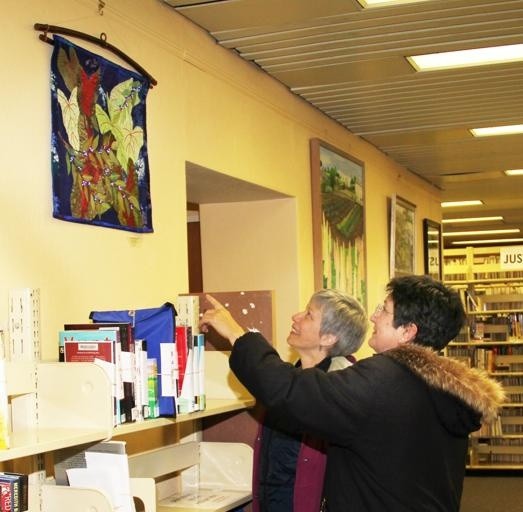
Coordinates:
[374,303,397,319]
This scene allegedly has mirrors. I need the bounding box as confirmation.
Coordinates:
[424,216,446,285]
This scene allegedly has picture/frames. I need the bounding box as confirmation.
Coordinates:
[386,192,419,282]
[307,137,367,314]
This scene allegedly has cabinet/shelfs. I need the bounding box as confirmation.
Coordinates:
[425,243,523,474]
[2,349,266,511]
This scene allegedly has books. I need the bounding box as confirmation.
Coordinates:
[446,254,523,465]
[57,292,210,428]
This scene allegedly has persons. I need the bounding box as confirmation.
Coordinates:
[252,286,368,512]
[200,274,508,512]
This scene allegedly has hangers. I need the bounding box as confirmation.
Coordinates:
[31,4,160,88]
